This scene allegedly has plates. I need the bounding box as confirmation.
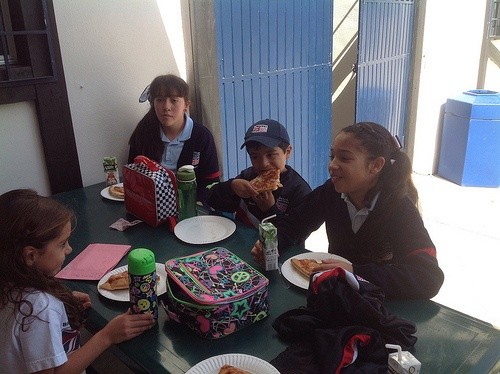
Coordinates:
[183,353,280,374]
[173,215,236,244]
[100,183,126,202]
[281,252,352,290]
[97,263,170,301]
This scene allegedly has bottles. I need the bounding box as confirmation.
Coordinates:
[127,248,158,321]
[177,165,198,222]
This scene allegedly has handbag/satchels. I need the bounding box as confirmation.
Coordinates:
[121,155,180,233]
[160,247,270,340]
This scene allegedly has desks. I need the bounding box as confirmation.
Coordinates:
[46,175,500,374]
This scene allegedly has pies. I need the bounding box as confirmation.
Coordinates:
[218,364,252,374]
[109,185,125,199]
[250,170,283,193]
[99,270,129,290]
[290,259,318,277]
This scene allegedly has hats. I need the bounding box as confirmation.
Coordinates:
[240,119,291,150]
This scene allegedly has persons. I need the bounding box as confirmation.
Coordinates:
[0,189,155,374]
[128,74,220,207]
[250,122,445,299]
[201,118,312,249]
